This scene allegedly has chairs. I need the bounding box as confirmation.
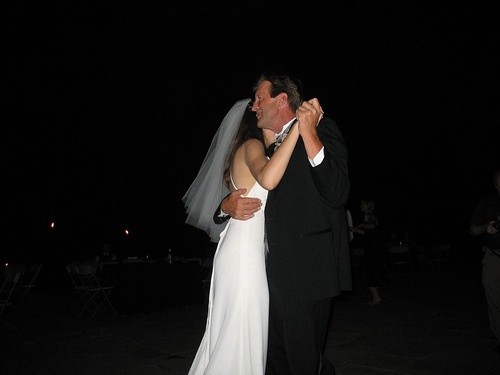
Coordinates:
[0,261,119,334]
